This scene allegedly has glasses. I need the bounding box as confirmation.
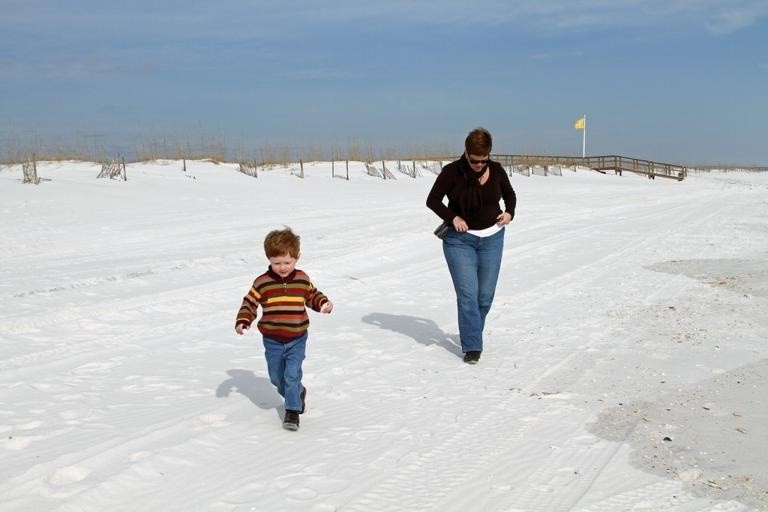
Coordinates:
[468,155,489,164]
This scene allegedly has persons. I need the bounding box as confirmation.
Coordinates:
[425,126,516,365]
[235,226,333,430]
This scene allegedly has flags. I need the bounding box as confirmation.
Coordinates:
[575,118,586,129]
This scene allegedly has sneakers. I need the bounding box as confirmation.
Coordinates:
[282,412,299,430]
[299,387,305,414]
[463,351,481,363]
